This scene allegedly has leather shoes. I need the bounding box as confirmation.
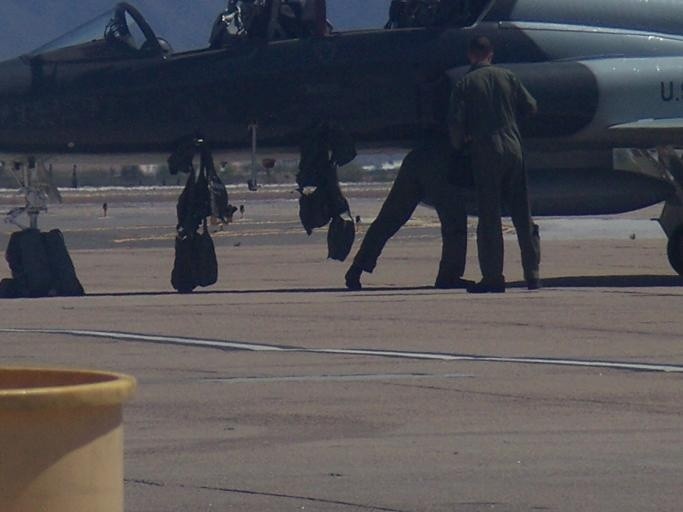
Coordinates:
[467,275,505,293]
[345,265,362,290]
[434,276,476,289]
[526,278,542,290]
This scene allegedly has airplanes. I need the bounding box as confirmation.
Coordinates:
[0,0,681,300]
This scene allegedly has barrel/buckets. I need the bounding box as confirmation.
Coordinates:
[1,367,138,508]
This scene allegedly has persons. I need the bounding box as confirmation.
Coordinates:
[445,35,543,295]
[342,141,476,291]
